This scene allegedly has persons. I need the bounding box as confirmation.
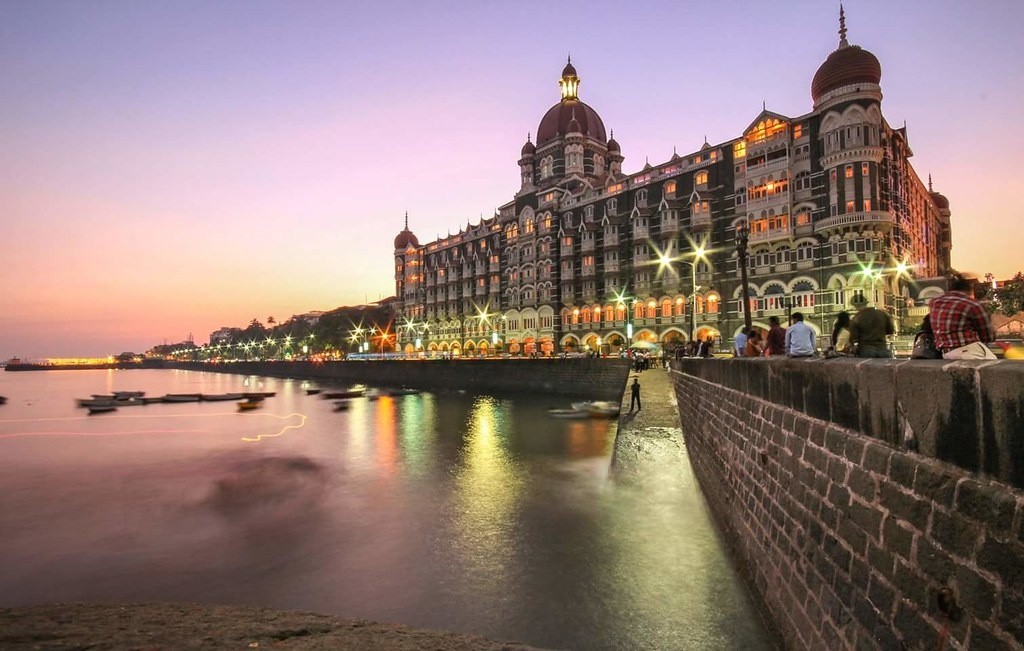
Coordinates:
[930,278,996,360]
[849,295,895,358]
[911,315,943,360]
[746,330,762,358]
[589,346,601,357]
[619,348,652,373]
[830,310,854,357]
[733,327,749,357]
[785,312,816,357]
[760,315,787,356]
[629,379,642,412]
[677,336,715,358]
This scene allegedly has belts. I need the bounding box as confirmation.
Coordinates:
[941,345,957,354]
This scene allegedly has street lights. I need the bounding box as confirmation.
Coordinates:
[734,227,753,330]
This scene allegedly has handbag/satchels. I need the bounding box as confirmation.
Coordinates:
[910,331,941,360]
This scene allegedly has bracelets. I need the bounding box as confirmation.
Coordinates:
[760,350,763,354]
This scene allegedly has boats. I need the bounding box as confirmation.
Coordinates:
[387,389,419,396]
[335,400,353,408]
[571,401,621,416]
[77,389,277,411]
[307,388,321,394]
[321,390,362,398]
[549,408,590,419]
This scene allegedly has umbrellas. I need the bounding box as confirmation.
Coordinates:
[628,339,657,351]
